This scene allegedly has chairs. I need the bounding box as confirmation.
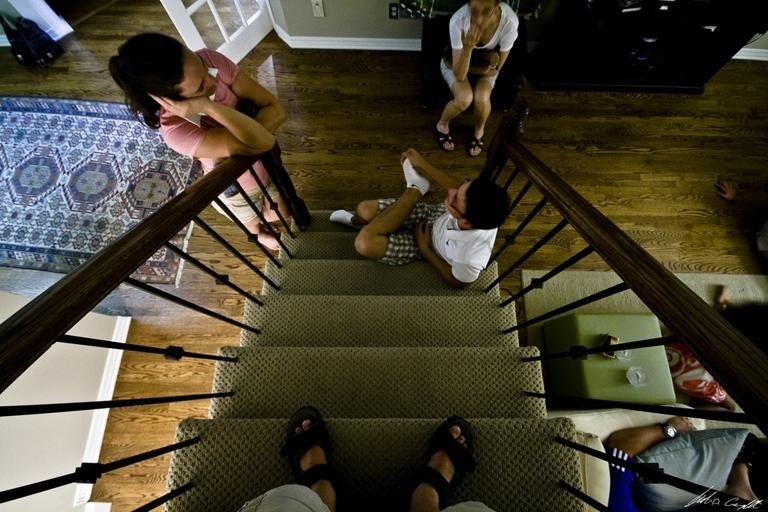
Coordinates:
[546,404,706,512]
[420,13,530,113]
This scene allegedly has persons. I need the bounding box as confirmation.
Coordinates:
[714,177,767,202]
[600,417,768,512]
[237,406,501,512]
[715,285,735,310]
[438,0,531,156]
[105,33,309,252]
[326,147,511,288]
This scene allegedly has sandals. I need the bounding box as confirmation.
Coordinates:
[407,416,474,508]
[285,406,335,492]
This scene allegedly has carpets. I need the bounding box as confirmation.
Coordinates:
[522,267,768,412]
[0,96,203,288]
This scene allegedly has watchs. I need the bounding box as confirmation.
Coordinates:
[656,422,677,439]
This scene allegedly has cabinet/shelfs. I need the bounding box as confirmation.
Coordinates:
[519,0,768,96]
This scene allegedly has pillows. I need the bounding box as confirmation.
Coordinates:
[633,428,748,512]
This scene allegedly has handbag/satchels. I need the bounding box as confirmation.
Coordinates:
[1,12,67,72]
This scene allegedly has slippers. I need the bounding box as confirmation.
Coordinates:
[469,134,484,157]
[436,124,454,152]
